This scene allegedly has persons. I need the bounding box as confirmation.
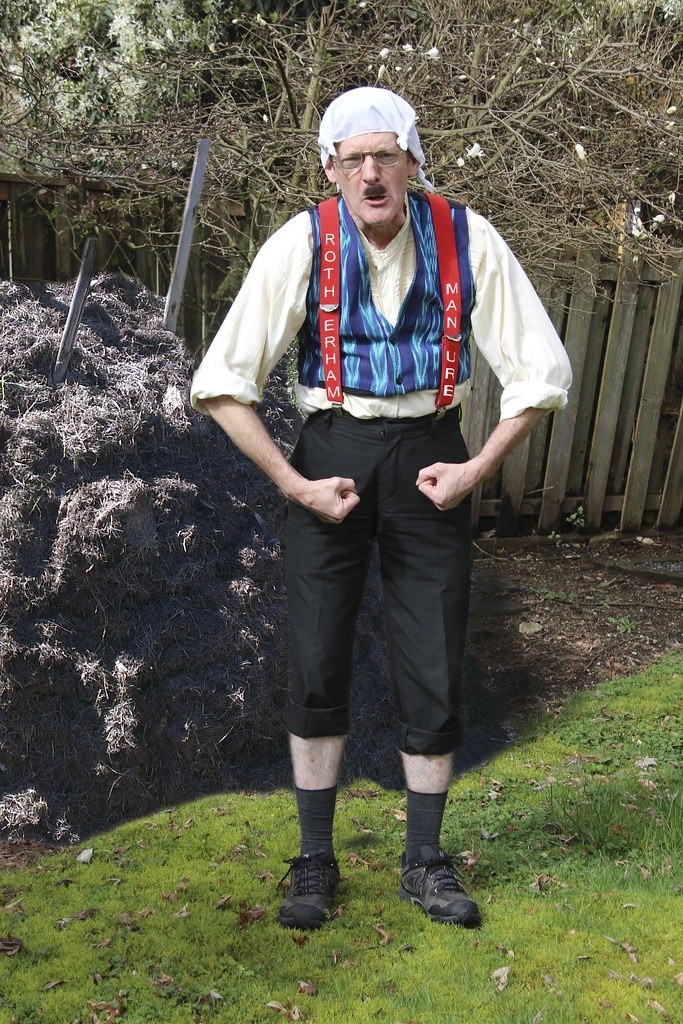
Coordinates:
[190,87,572,931]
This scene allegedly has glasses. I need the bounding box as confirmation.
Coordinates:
[331,147,406,172]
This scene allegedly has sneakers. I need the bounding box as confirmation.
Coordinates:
[276,853,340,930]
[398,851,482,929]
